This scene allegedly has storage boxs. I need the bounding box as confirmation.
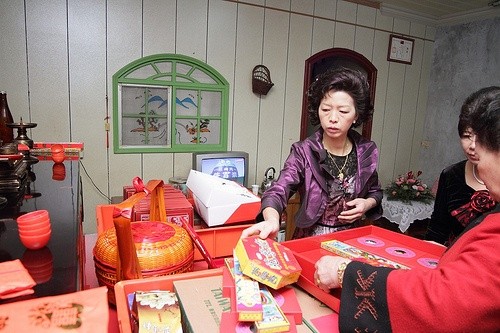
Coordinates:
[93,169,448,333]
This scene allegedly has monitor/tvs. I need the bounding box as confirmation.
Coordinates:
[192,151,249,188]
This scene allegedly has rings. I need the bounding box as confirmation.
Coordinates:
[316,279,320,284]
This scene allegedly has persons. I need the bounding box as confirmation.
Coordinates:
[422,85,500,248]
[314,89,500,333]
[241,67,384,241]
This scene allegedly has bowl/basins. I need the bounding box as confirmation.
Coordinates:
[52,163,66,180]
[20,246,54,285]
[51,144,65,163]
[16,210,51,251]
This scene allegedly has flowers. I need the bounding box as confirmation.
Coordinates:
[385,170,436,206]
[451,190,495,228]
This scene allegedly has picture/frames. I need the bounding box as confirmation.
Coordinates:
[387,34,415,65]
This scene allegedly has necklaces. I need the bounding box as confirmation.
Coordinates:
[472,163,484,185]
[323,141,349,184]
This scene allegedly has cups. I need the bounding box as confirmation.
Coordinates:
[251,185,259,196]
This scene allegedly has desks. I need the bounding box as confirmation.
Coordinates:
[382,193,435,233]
[0,160,83,304]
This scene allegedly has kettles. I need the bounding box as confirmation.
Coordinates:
[261,167,277,192]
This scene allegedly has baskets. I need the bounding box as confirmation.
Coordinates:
[93,179,195,306]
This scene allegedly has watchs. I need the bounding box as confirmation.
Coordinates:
[337,260,352,288]
